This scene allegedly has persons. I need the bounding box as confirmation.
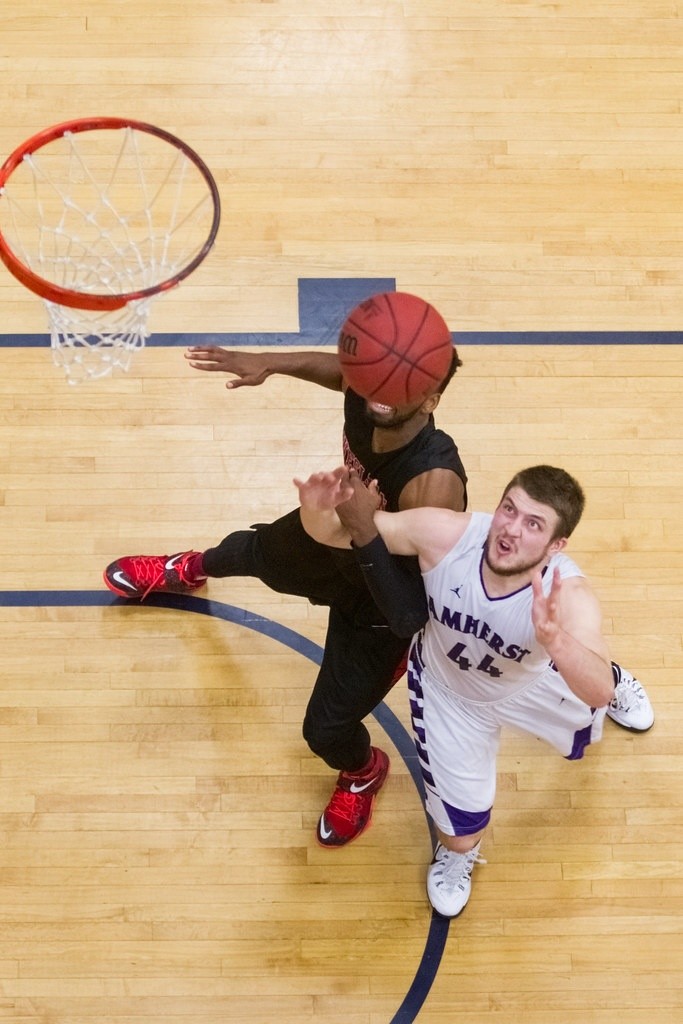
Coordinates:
[104,348,468,847]
[294,466,654,919]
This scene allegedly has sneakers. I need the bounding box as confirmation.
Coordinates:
[426,838,487,919]
[103,549,208,602]
[316,746,389,849]
[606,660,655,732]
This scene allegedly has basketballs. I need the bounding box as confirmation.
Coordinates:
[334,290,456,407]
